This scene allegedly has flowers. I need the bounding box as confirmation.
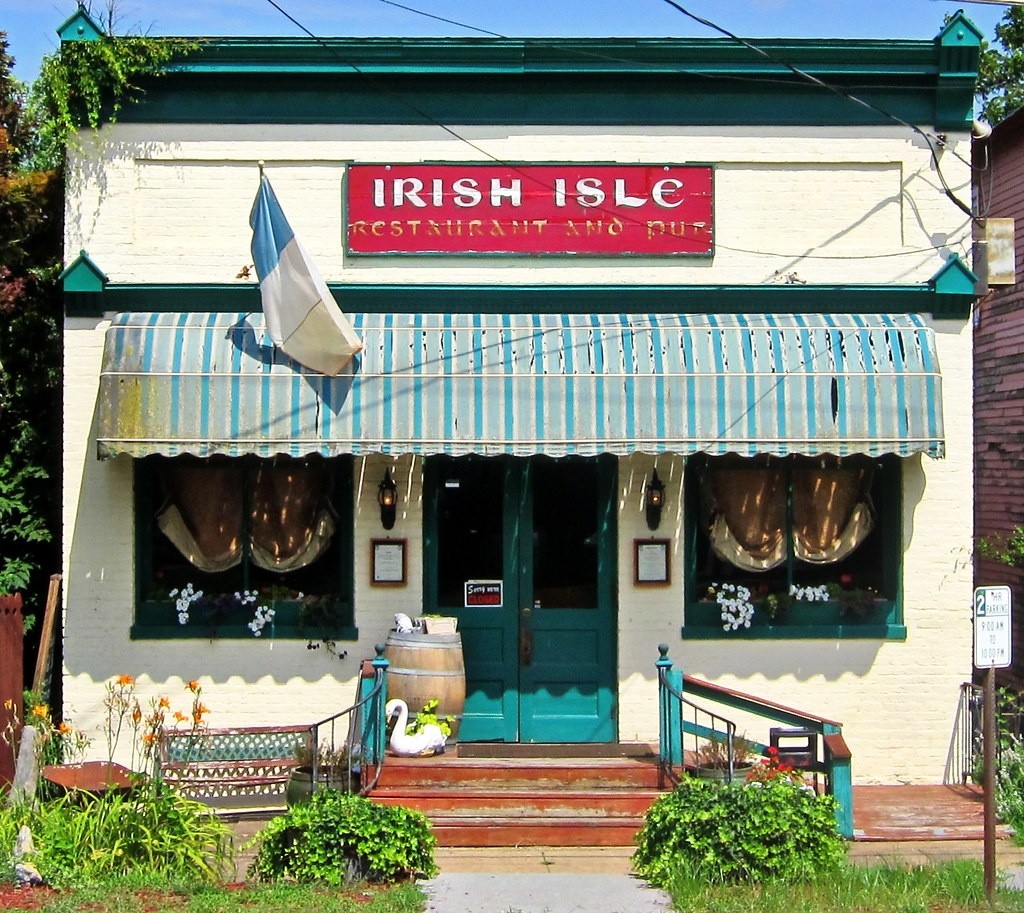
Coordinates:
[229,590,277,638]
[168,583,204,626]
[706,582,756,632]
[789,584,878,626]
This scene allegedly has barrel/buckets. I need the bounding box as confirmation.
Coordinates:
[383,629,466,753]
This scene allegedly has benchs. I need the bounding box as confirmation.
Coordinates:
[159,724,314,822]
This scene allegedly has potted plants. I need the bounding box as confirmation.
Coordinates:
[286,729,372,810]
[688,728,760,781]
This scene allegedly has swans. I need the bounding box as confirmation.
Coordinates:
[385,698,448,757]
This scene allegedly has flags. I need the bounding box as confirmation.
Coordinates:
[250,177,363,380]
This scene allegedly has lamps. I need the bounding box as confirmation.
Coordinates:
[645,468,667,530]
[377,467,399,530]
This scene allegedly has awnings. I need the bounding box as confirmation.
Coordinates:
[96,310,950,460]
